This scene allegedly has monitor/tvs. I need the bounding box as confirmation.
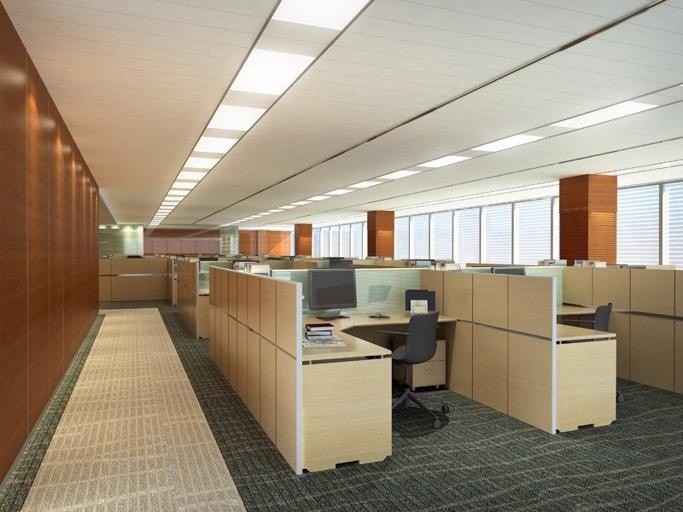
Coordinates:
[198,256,218,272]
[309,268,357,321]
[491,266,526,275]
[232,260,259,270]
[409,258,436,268]
[321,257,354,269]
[621,265,647,269]
[127,256,142,258]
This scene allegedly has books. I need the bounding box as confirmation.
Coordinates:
[303,334,335,341]
[233,258,272,277]
[304,321,336,331]
[307,332,335,336]
[302,341,346,348]
